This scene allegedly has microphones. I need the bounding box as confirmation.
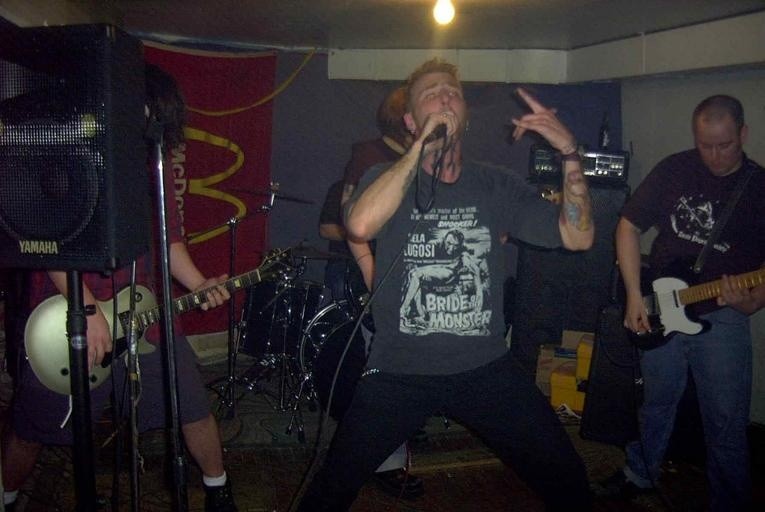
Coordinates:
[425,122,448,146]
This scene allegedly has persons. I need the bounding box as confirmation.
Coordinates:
[600,94,765,512]
[298,58,593,511]
[0,76,237,512]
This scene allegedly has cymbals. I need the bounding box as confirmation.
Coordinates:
[253,241,345,262]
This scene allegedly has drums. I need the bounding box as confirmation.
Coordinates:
[234,277,327,363]
[301,298,372,420]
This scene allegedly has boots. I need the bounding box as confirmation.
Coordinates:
[375,468,424,500]
[201,478,238,512]
[607,469,656,497]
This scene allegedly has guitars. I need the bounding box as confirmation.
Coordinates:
[617,264,761,350]
[23,247,295,396]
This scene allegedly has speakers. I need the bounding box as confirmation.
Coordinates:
[513,182,637,368]
[0,22,157,279]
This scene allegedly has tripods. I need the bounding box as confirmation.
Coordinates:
[250,293,319,415]
[203,230,292,422]
